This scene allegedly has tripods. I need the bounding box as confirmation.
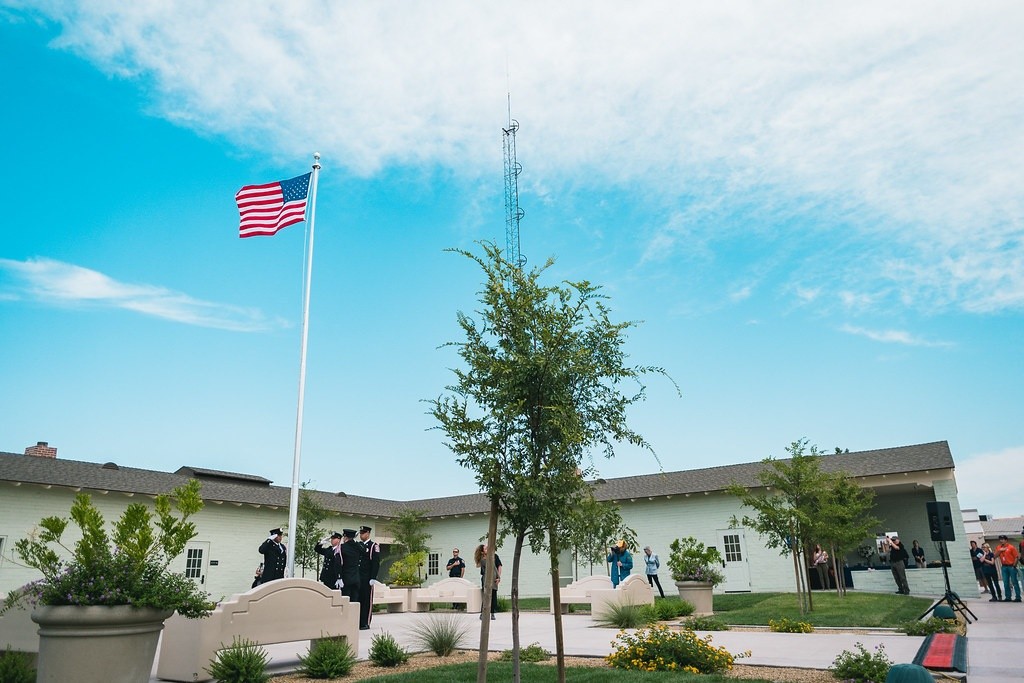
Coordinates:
[918,540,978,625]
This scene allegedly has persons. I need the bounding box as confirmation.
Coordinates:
[314,526,380,630]
[979,543,1002,602]
[970,541,991,594]
[886,536,909,595]
[607,540,633,589]
[994,535,1024,602]
[258,528,287,582]
[814,544,830,589]
[912,539,926,568]
[644,546,665,598]
[446,548,465,609]
[474,544,502,620]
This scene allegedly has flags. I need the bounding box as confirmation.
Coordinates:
[235,172,313,238]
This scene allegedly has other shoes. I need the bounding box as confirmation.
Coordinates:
[895,590,903,594]
[480,615,483,620]
[491,615,496,620]
[981,590,988,594]
[360,625,369,630]
[989,590,992,594]
[989,597,996,602]
[1012,599,1022,602]
[905,591,910,594]
[1002,598,1011,602]
[997,598,1002,601]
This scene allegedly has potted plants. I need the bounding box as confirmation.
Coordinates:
[10,477,206,683]
[385,553,427,611]
[666,537,725,618]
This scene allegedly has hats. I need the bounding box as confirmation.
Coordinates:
[891,536,898,541]
[332,533,342,539]
[343,529,357,537]
[999,535,1008,541]
[617,540,626,548]
[360,526,372,531]
[270,528,283,535]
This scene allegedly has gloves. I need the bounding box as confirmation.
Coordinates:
[270,534,278,540]
[320,537,330,545]
[369,579,374,586]
[336,579,344,589]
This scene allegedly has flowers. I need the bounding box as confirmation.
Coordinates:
[0,562,225,621]
[671,567,727,589]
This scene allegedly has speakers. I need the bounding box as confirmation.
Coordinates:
[926,501,955,542]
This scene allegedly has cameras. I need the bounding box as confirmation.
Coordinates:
[611,546,620,554]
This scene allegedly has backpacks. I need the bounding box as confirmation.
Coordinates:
[903,549,909,568]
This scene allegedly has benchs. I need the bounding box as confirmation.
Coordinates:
[372,579,408,613]
[590,575,656,621]
[155,578,361,683]
[550,575,613,615]
[410,578,483,614]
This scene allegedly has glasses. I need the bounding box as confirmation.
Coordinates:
[453,551,458,553]
